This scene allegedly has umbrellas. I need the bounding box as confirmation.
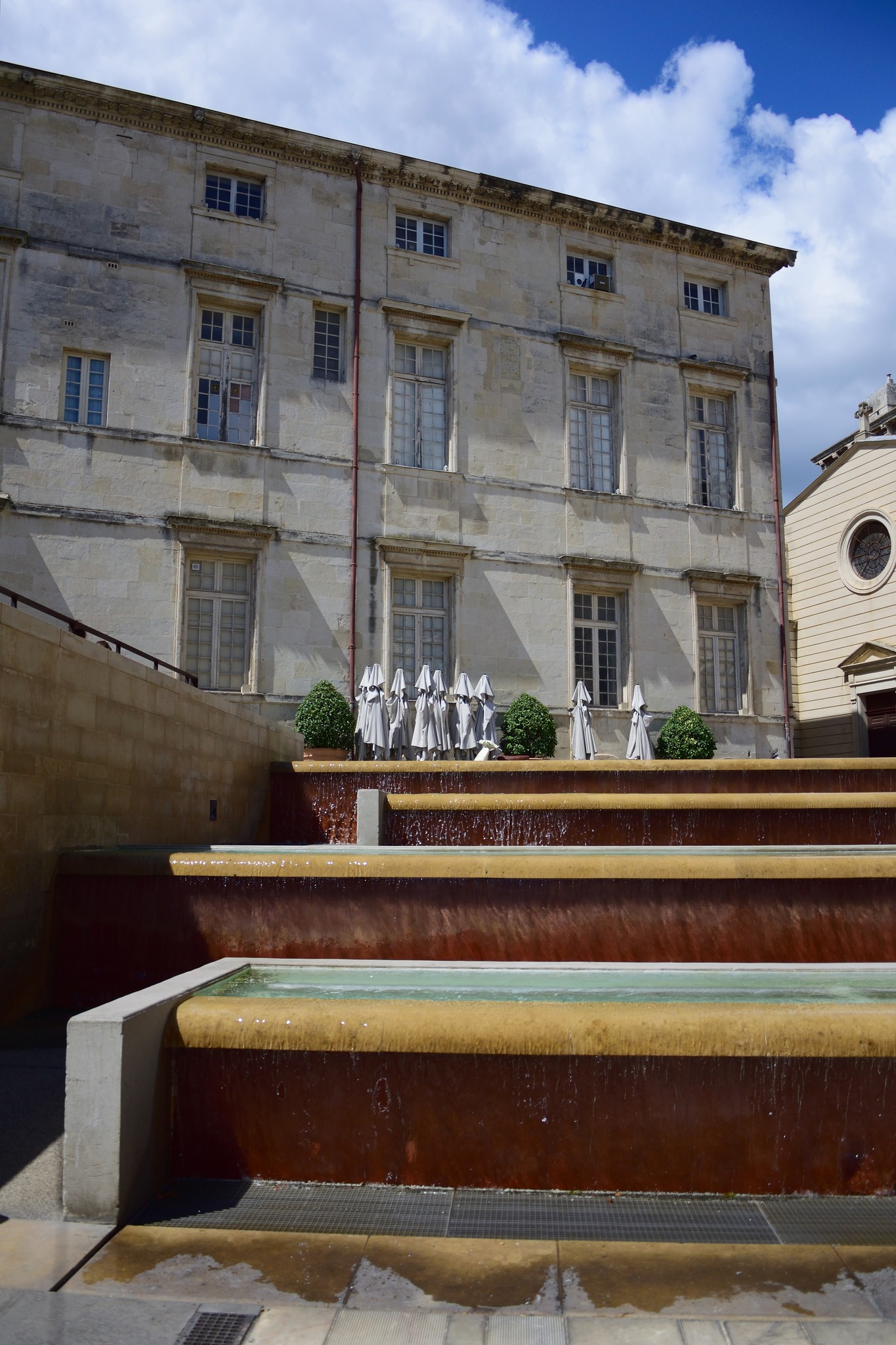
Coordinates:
[384,667,417,761]
[354,662,390,761]
[433,670,452,761]
[451,672,478,760]
[627,685,659,760]
[570,680,599,760]
[474,674,503,760]
[411,664,438,761]
